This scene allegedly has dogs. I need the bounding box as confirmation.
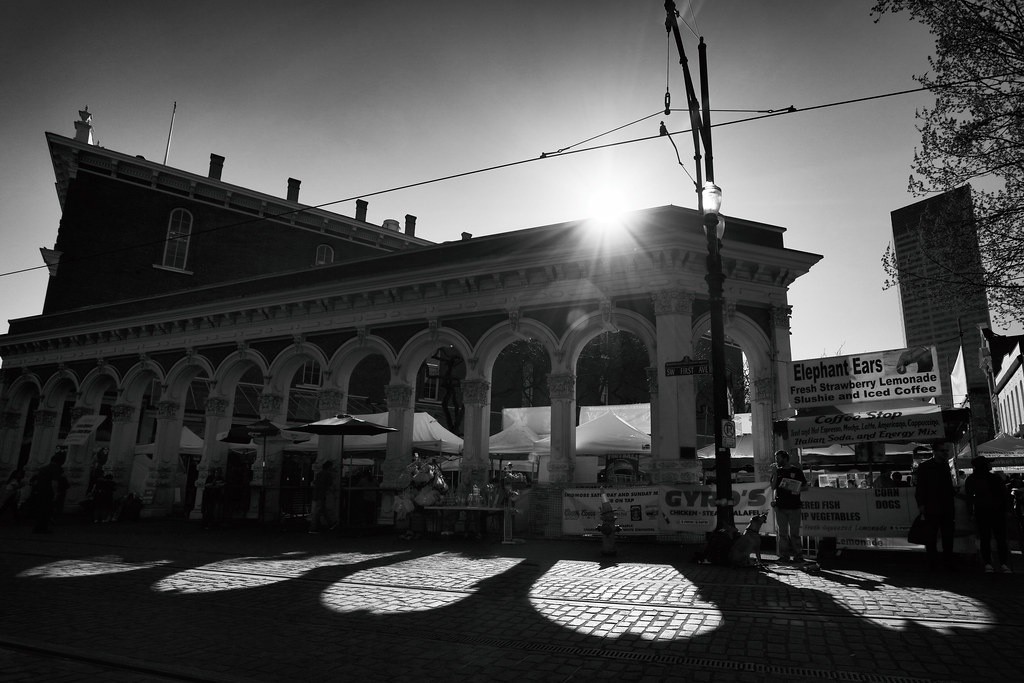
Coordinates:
[729,515,768,574]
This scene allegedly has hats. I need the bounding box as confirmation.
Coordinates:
[215,467,223,474]
[972,456,996,466]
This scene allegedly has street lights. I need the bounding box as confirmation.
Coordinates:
[697,179,746,566]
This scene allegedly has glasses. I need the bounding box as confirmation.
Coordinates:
[936,449,949,452]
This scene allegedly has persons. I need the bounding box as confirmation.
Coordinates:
[28,466,71,534]
[308,458,339,533]
[91,474,119,524]
[769,450,808,563]
[847,438,1024,574]
[201,466,226,529]
[0,470,22,527]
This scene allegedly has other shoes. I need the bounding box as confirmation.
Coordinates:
[329,523,337,530]
[794,554,803,563]
[103,512,120,522]
[984,563,994,573]
[998,564,1013,575]
[776,557,790,566]
[307,530,320,534]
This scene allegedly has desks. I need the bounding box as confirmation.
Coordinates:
[423,506,517,544]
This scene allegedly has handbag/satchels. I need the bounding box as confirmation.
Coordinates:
[908,513,937,545]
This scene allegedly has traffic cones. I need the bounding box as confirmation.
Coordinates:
[598,486,617,515]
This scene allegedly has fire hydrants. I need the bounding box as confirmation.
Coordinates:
[597,510,622,556]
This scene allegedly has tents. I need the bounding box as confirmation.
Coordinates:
[135,411,754,483]
[948,433,1024,485]
